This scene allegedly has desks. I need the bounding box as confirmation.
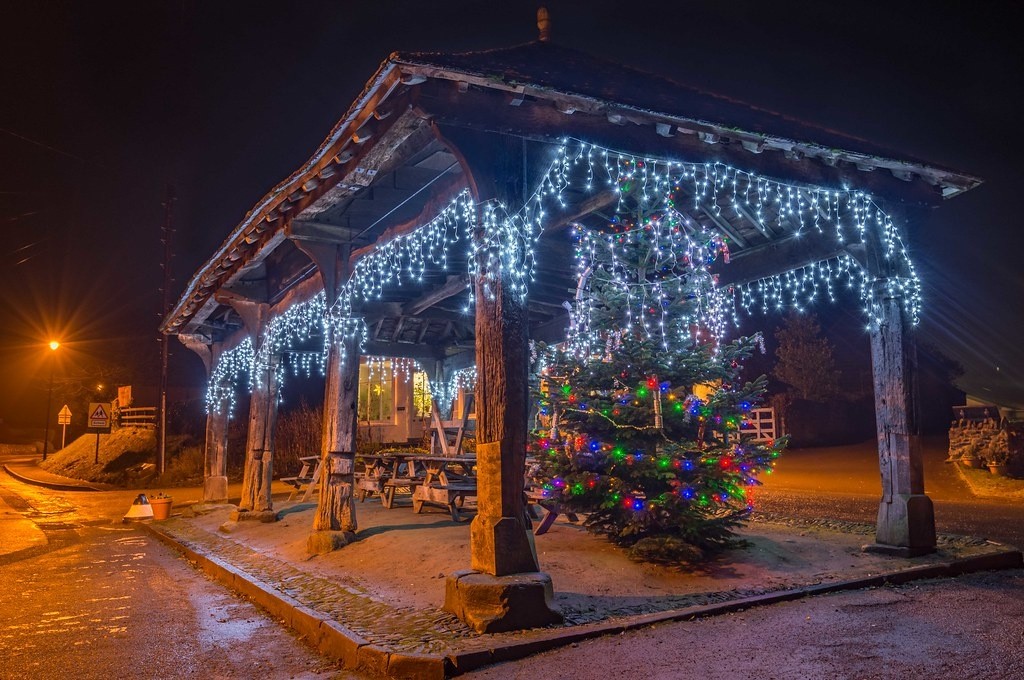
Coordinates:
[382,452,448,511]
[403,457,476,521]
[354,454,422,505]
[288,456,321,503]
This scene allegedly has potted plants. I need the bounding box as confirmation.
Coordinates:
[961,445,979,467]
[148,492,174,520]
[986,443,1009,475]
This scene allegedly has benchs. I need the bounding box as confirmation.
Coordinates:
[279,469,550,503]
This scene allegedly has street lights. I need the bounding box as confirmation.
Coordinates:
[43,341,59,460]
